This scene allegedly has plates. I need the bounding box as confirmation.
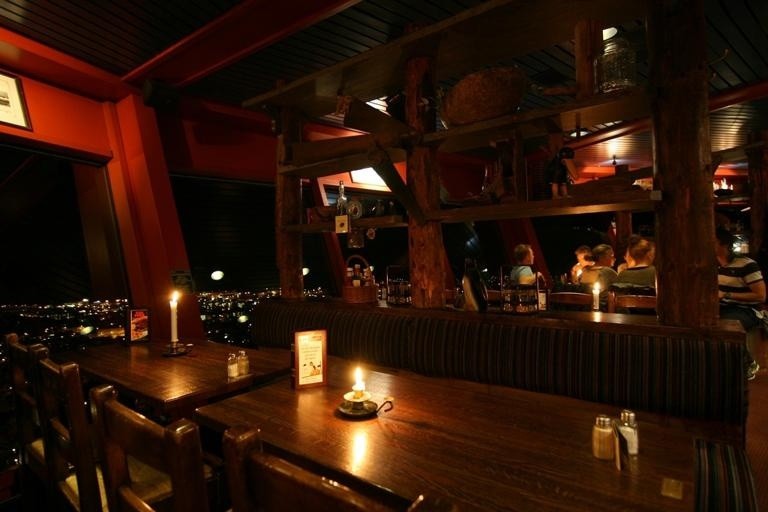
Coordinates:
[159,343,194,357]
[337,400,378,417]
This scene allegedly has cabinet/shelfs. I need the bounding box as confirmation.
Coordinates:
[244,1,725,326]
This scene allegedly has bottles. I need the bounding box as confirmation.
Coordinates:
[227,353,239,379]
[344,263,361,288]
[560,182,567,196]
[592,413,614,460]
[236,350,250,375]
[336,180,348,215]
[386,279,411,305]
[614,408,640,459]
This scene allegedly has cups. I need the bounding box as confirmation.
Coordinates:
[560,272,567,284]
[552,182,558,197]
[501,292,538,314]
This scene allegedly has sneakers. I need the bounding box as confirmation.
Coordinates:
[747,360,760,378]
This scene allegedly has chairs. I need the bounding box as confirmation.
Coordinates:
[0,287,766,512]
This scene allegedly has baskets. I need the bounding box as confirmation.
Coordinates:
[341,255,379,305]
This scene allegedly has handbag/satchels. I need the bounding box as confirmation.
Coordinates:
[720,298,767,330]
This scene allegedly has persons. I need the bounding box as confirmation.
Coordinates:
[510,244,546,287]
[578,245,618,293]
[617,234,656,296]
[571,246,595,285]
[714,226,766,380]
